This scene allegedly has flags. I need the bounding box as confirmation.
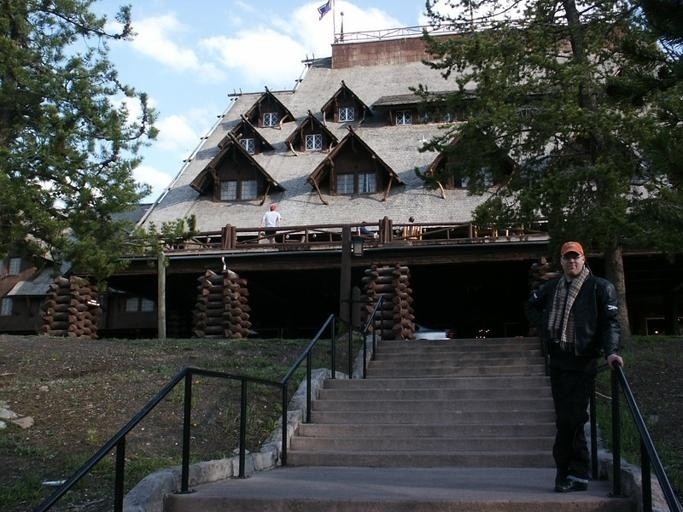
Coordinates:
[316,0,333,21]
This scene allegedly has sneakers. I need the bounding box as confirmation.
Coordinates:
[554,472,588,492]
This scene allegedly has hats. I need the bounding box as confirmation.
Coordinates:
[560,241,584,257]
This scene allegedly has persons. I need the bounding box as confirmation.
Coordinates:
[256,230,271,244]
[401,216,421,241]
[359,221,371,233]
[536,242,626,494]
[258,203,282,243]
